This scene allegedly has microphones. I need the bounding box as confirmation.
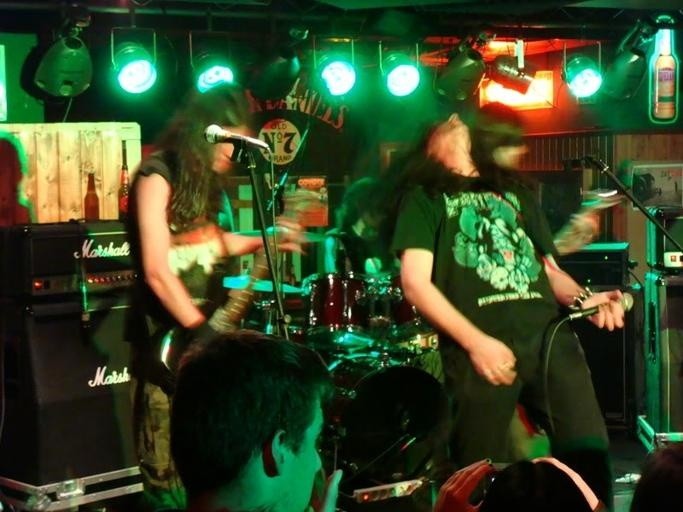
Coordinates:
[568,292,635,321]
[202,123,271,149]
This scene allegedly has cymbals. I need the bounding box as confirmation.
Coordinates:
[231,228,322,245]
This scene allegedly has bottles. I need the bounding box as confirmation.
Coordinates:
[84,172,99,220]
[599,12,679,124]
[117,163,131,220]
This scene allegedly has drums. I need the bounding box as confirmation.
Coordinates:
[366,269,416,323]
[321,350,451,495]
[314,275,369,327]
[222,276,310,336]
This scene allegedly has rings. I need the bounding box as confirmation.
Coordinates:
[480,372,493,382]
[490,370,501,376]
[498,361,508,372]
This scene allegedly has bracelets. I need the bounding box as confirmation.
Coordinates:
[566,285,593,311]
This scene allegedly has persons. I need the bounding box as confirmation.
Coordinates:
[476,106,603,266]
[160,327,345,511]
[626,438,681,511]
[381,110,627,511]
[122,76,309,512]
[0,134,34,227]
[430,446,616,510]
[329,141,414,260]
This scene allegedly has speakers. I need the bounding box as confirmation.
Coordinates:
[556,240,636,423]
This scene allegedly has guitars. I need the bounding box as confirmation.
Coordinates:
[146,175,322,378]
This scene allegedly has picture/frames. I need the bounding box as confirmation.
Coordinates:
[631,165,683,210]
